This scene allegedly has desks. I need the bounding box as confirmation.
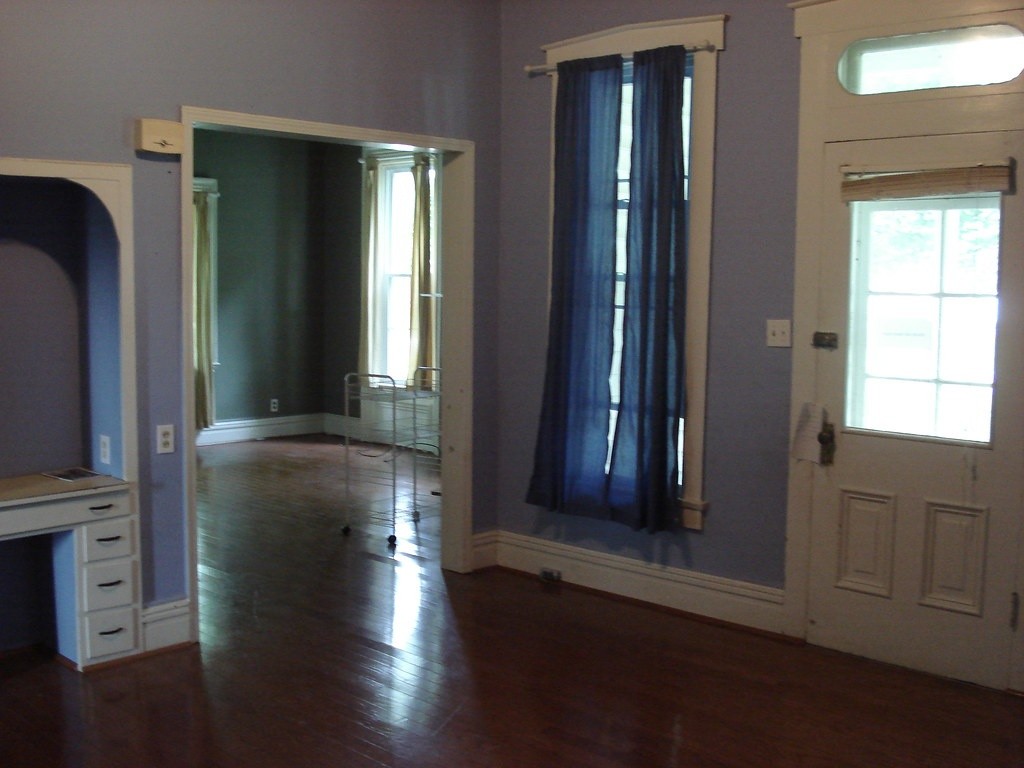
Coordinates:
[0,466,145,674]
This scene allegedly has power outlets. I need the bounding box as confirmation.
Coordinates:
[270,398,279,412]
[100,434,110,465]
[156,425,174,454]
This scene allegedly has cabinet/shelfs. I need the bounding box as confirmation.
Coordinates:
[343,367,442,543]
